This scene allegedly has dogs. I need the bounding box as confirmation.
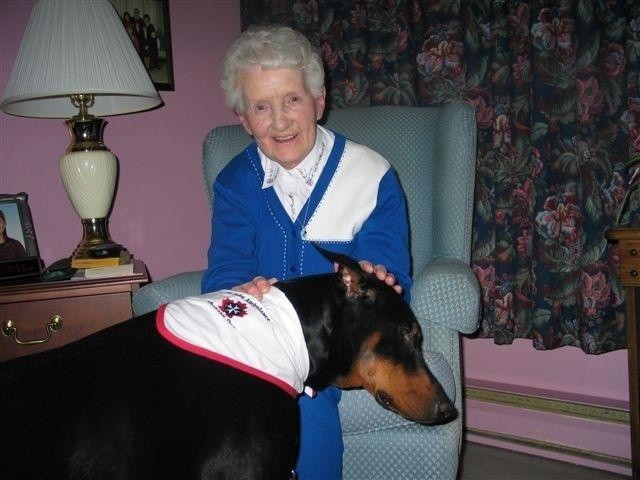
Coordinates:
[0,240,459,480]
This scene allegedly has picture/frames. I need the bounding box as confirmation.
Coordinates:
[110,2,176,91]
[1,192,44,282]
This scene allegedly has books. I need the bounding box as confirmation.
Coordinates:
[70,254,143,282]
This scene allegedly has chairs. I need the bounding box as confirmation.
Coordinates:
[132,100,485,479]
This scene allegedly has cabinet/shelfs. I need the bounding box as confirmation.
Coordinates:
[0,260,151,364]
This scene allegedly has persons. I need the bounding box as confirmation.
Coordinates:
[201,21,412,480]
[1,210,26,259]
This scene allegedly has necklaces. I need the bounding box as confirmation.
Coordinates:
[275,179,311,237]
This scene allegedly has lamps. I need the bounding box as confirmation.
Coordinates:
[1,0,165,269]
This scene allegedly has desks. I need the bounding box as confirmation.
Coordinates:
[605,228,640,477]
